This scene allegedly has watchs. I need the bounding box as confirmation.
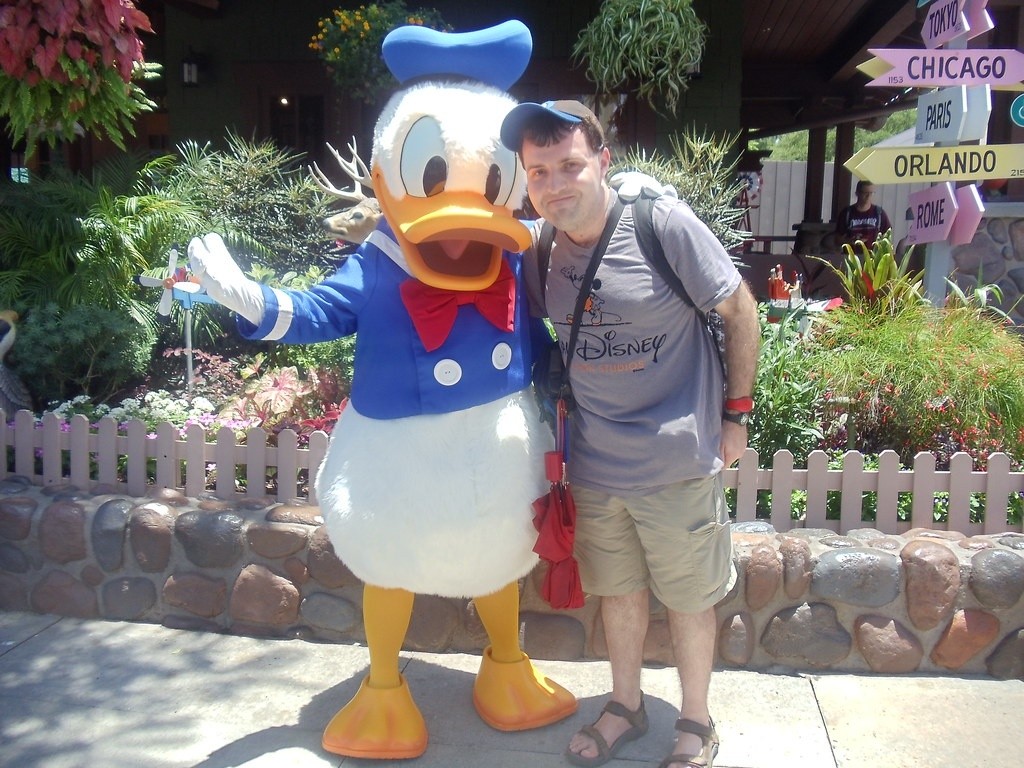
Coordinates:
[723,413,749,427]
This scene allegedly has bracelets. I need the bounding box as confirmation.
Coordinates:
[727,396,752,414]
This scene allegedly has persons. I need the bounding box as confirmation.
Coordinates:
[502,100,757,768]
[835,180,892,274]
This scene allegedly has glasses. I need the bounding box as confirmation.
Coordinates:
[862,191,874,196]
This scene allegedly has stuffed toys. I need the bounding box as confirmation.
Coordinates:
[188,19,684,759]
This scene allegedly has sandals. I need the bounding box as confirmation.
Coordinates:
[567,689,650,765]
[658,715,720,768]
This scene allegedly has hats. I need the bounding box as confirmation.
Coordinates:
[500,99,604,152]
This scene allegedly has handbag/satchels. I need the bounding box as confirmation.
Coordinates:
[532,340,576,462]
